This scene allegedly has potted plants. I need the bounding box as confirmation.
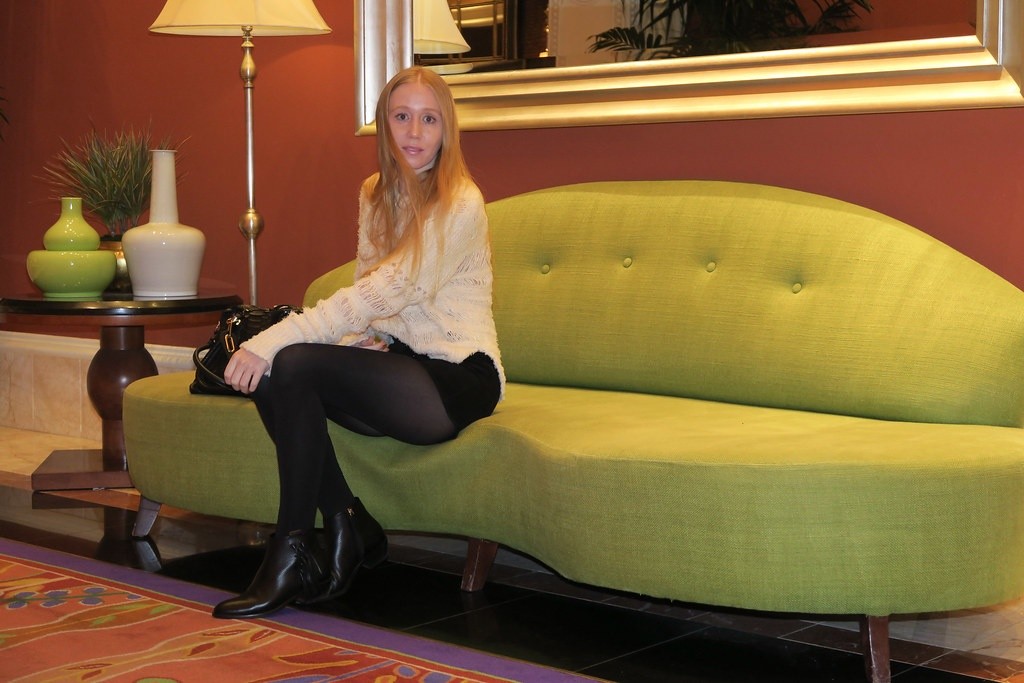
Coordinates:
[39,124,192,290]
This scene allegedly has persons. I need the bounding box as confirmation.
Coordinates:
[213,67,506,619]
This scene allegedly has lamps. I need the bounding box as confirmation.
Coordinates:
[412,0,472,55]
[148,0,332,305]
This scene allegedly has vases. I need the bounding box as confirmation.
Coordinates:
[121,149,205,297]
[25,196,118,298]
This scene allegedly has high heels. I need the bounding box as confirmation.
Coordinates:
[295,496,390,606]
[212,527,331,619]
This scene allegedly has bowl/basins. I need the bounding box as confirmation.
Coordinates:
[26,249,117,302]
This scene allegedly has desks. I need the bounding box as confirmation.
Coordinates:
[0,290,242,491]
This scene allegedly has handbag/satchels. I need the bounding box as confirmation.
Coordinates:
[190,302,303,397]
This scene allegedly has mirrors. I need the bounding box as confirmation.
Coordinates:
[353,0,1024,139]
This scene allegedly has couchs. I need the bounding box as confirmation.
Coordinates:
[124,181,1023,683]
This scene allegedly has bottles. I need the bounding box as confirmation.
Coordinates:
[43,197,101,251]
[120,149,207,300]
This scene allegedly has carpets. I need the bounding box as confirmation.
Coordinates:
[0,535,617,683]
